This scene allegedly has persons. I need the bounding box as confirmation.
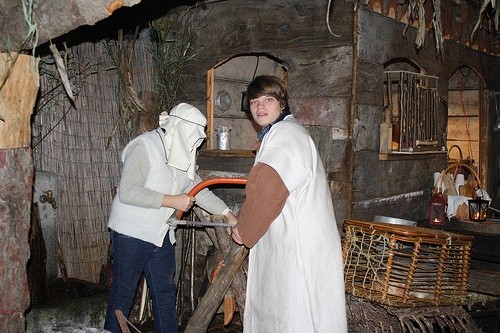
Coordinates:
[232,74,348,333]
[103,103,242,333]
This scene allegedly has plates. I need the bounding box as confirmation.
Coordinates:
[214,89,232,111]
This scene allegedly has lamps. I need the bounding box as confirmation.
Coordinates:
[467,186,490,222]
[428,194,447,226]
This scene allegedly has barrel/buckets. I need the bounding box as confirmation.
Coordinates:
[363,215,417,263]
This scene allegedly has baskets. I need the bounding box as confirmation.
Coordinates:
[433,146,483,221]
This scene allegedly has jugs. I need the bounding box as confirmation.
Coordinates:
[216,125,232,151]
